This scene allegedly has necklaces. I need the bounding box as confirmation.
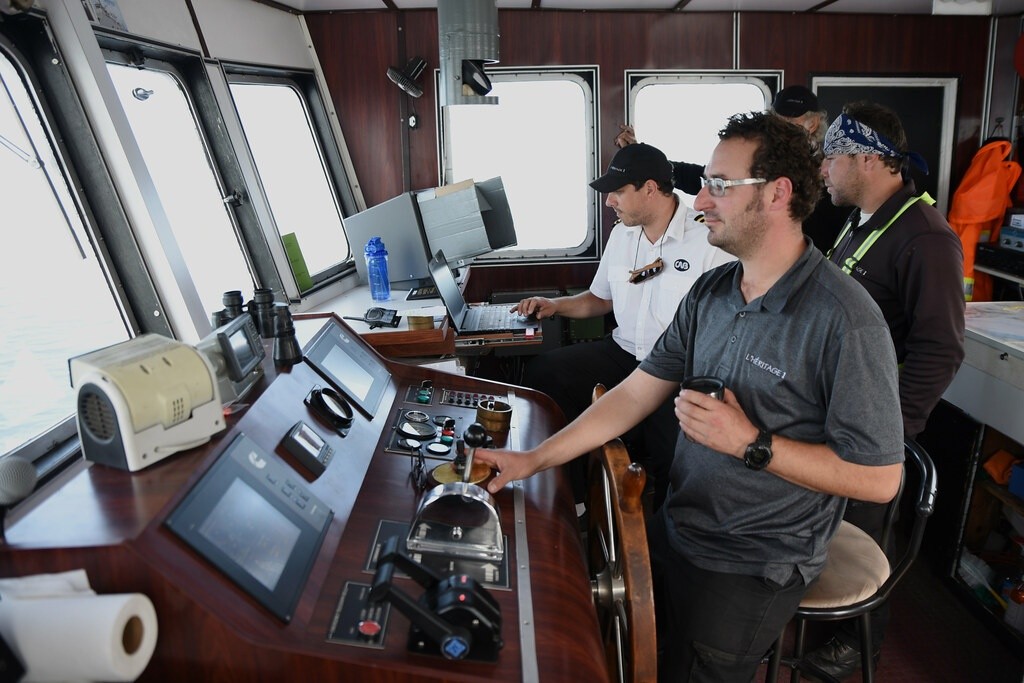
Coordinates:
[627,196,677,285]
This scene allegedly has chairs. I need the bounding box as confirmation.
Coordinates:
[766,435,937,683]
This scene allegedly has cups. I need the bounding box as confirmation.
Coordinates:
[680,376,724,444]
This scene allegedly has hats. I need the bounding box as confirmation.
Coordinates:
[589,142,672,193]
[771,85,818,117]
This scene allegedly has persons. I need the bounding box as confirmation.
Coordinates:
[796,101,966,683]
[467,112,906,683]
[511,145,740,509]
[770,87,857,250]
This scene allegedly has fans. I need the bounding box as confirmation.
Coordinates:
[384,55,429,99]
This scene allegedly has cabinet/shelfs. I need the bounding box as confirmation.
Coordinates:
[947,421,1024,660]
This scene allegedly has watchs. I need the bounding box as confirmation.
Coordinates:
[744,429,773,471]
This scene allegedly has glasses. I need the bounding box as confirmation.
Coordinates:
[699,176,767,196]
[629,257,664,284]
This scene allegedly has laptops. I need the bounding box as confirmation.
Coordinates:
[427,249,538,338]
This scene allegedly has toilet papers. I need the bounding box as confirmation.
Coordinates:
[0,592,159,683]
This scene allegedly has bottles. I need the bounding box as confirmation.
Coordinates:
[364,237,392,303]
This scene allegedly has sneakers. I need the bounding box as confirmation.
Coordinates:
[798,634,881,681]
